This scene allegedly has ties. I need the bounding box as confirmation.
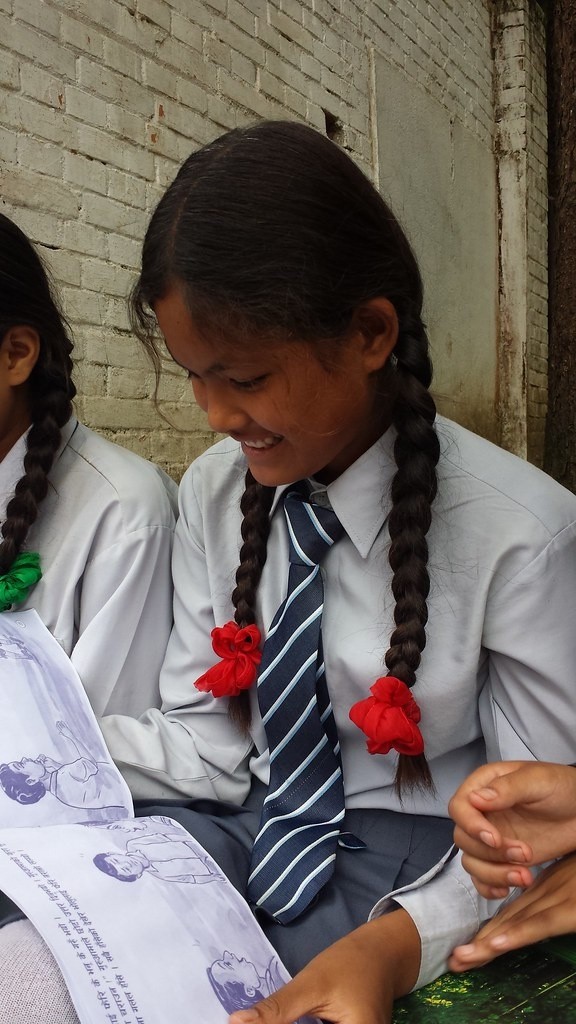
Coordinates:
[251,492,353,925]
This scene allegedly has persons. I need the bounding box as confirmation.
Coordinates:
[1,119,576,1023]
[442,759,576,973]
[1,213,178,728]
